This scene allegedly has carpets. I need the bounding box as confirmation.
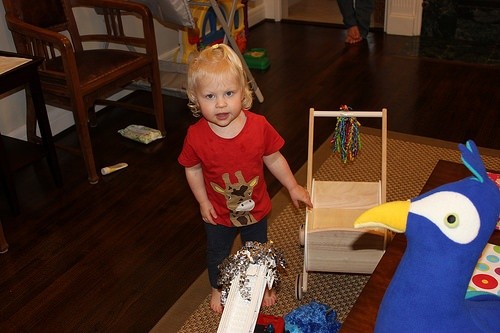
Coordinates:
[146,125,500,332]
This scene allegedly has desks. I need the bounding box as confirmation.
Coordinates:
[329,156,500,333]
[0,49,70,257]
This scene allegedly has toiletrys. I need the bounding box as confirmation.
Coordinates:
[101,161,128,176]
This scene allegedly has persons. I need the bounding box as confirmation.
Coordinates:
[177,42,314,314]
[335,0,374,44]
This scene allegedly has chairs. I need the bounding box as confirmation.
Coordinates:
[1,0,167,185]
